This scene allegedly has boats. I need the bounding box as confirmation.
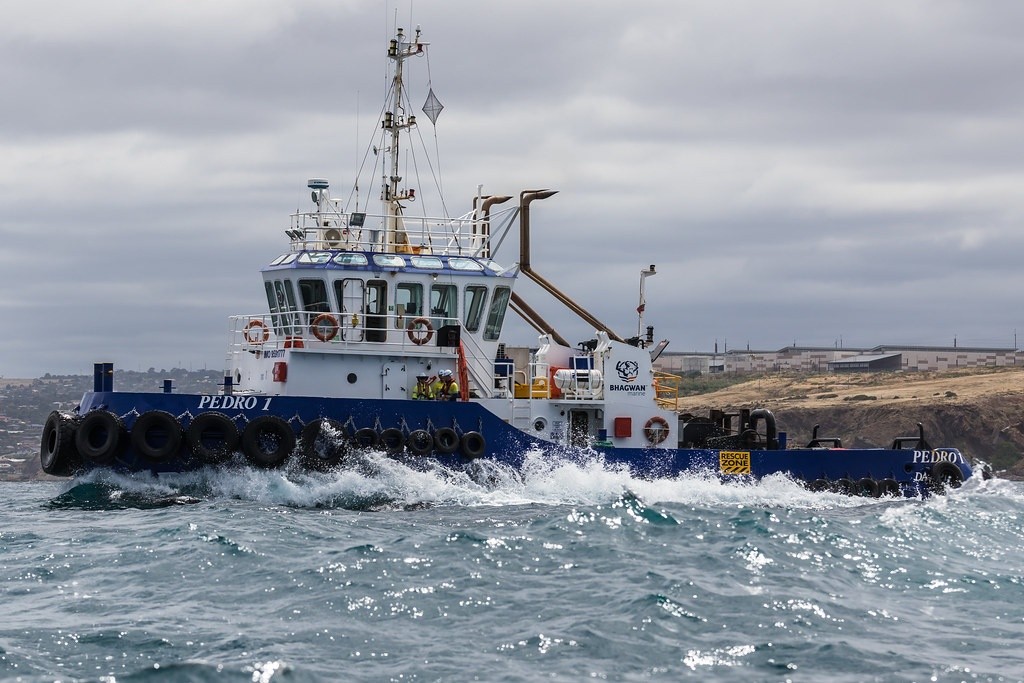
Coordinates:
[36,26,975,499]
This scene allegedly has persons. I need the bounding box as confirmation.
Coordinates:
[412,369,458,401]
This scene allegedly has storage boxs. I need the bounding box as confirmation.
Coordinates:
[515,384,550,397]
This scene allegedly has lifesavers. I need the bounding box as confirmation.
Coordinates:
[354,427,381,454]
[811,478,900,499]
[184,411,240,464]
[408,318,434,344]
[128,408,183,460]
[931,460,964,489]
[244,321,269,345]
[310,315,339,342]
[461,430,487,458]
[380,426,405,455]
[405,429,432,456]
[40,409,75,477]
[72,409,128,464]
[295,417,352,473]
[644,416,671,443]
[240,413,294,470]
[432,425,459,454]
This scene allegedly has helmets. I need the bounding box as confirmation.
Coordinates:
[415,372,429,378]
[438,369,444,376]
[442,369,453,377]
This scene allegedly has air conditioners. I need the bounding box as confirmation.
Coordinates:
[322,228,351,250]
